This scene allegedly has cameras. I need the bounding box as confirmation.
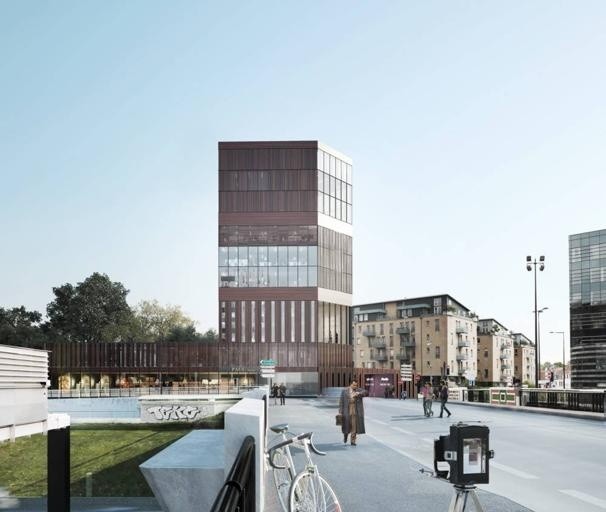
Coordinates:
[433,420,495,486]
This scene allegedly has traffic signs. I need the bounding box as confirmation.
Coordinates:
[261,359,278,366]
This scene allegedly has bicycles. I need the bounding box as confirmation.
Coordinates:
[266,423,341,512]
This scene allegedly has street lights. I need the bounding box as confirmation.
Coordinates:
[550,331,566,388]
[527,255,548,389]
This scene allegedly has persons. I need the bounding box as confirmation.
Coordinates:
[425,382,435,418]
[278,382,286,405]
[338,379,370,448]
[271,383,279,398]
[422,381,428,416]
[437,379,451,418]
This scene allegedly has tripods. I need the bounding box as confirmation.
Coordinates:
[448,485,484,512]
